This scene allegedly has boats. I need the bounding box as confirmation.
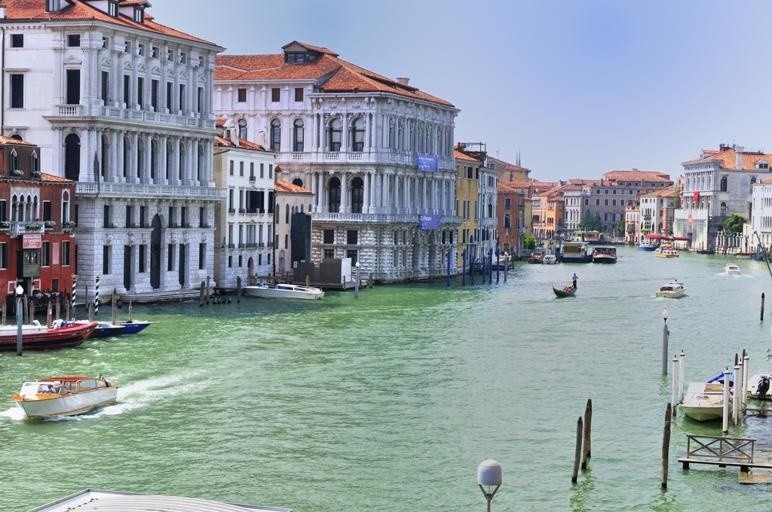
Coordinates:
[12,375,117,421]
[725,263,741,275]
[244,283,325,299]
[656,279,686,298]
[0,320,151,349]
[553,285,577,297]
[637,237,679,258]
[528,230,617,264]
[679,367,733,422]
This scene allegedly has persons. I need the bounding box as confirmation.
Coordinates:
[573,273,579,288]
